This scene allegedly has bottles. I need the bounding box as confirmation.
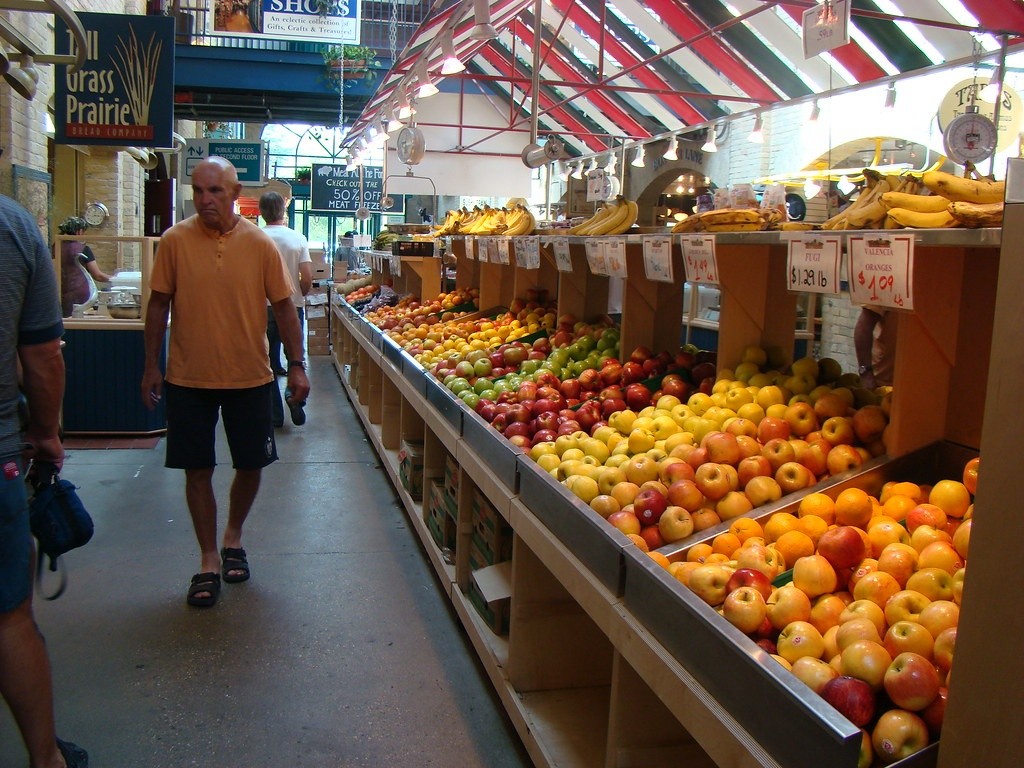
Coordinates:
[696,177,712,212]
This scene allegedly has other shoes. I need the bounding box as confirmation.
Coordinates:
[285,391,306,426]
[278,365,287,374]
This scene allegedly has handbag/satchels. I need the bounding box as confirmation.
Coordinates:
[28,469,95,572]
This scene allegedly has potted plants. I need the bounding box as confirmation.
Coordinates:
[202,121,225,139]
[321,45,381,94]
[298,169,310,184]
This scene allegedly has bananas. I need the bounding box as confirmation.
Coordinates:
[432,204,535,237]
[670,208,815,234]
[567,194,638,237]
[819,169,1004,227]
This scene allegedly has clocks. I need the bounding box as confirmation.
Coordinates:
[84,202,109,227]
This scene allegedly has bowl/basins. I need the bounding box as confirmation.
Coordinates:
[384,224,430,235]
[99,291,121,301]
[133,294,143,304]
[110,286,139,300]
[107,303,142,319]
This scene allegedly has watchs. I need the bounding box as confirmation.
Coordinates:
[859,365,872,374]
[288,361,306,368]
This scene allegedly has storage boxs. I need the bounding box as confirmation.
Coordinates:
[400,439,423,501]
[303,249,330,355]
[429,454,513,635]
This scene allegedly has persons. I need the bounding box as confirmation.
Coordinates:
[336,231,357,268]
[854,307,897,385]
[52,217,110,316]
[141,155,309,608]
[259,192,313,427]
[0,192,90,768]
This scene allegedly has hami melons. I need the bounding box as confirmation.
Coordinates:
[337,277,373,295]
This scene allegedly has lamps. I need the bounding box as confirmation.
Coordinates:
[560,113,763,181]
[346,0,499,173]
[885,82,896,108]
[805,98,820,121]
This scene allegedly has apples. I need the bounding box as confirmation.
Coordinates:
[687,458,982,766]
[366,292,718,456]
[346,284,378,304]
[532,345,894,546]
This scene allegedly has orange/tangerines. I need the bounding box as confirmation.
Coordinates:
[624,481,919,587]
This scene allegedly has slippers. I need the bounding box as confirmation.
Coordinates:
[54,736,87,768]
[220,546,251,584]
[186,572,221,608]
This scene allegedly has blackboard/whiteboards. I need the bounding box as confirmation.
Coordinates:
[309,163,385,213]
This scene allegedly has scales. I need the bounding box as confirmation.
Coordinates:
[599,137,620,204]
[339,206,371,246]
[380,83,437,234]
[943,24,998,180]
